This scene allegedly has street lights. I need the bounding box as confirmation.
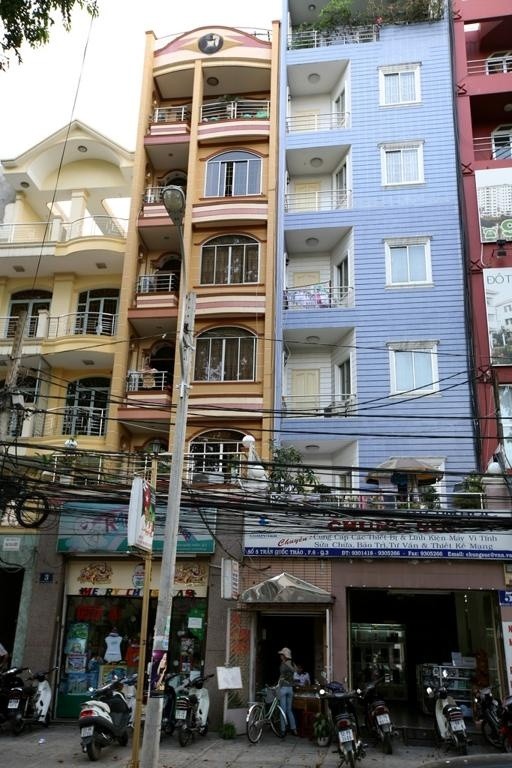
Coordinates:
[138,184,196,763]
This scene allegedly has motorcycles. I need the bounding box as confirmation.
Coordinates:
[426,670,471,756]
[79,670,215,762]
[2,665,59,735]
[473,683,512,752]
[318,677,400,768]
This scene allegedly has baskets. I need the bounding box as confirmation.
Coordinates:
[262,686,280,703]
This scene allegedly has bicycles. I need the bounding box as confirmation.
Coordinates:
[246,677,289,744]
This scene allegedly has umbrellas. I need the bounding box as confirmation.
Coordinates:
[366,456,444,508]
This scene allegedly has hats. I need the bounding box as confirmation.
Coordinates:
[278,647,292,659]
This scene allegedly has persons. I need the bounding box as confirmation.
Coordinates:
[0,643,9,672]
[293,664,312,688]
[205,357,226,381]
[268,647,298,738]
[138,362,160,390]
